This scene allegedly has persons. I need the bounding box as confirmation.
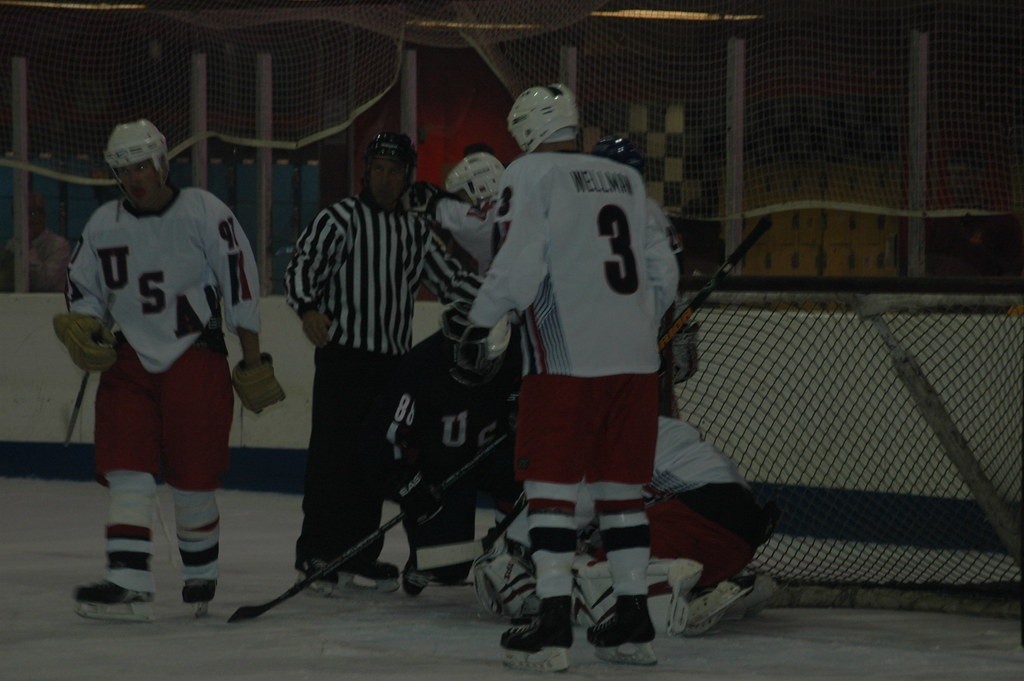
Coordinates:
[0,191,72,292]
[435,84,678,672]
[285,134,776,636]
[52,118,287,623]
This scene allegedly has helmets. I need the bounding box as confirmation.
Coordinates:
[590,135,648,177]
[444,151,509,211]
[104,119,170,179]
[506,82,579,157]
[363,130,415,180]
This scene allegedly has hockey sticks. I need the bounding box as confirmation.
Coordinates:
[414,213,775,575]
[60,293,119,451]
[226,409,515,626]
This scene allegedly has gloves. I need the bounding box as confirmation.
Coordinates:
[440,296,514,388]
[672,320,701,383]
[231,352,285,414]
[53,312,117,373]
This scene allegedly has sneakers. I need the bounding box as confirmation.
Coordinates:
[71,584,158,622]
[676,567,775,637]
[586,594,657,665]
[296,555,400,598]
[500,595,574,672]
[182,578,216,618]
[402,549,428,596]
